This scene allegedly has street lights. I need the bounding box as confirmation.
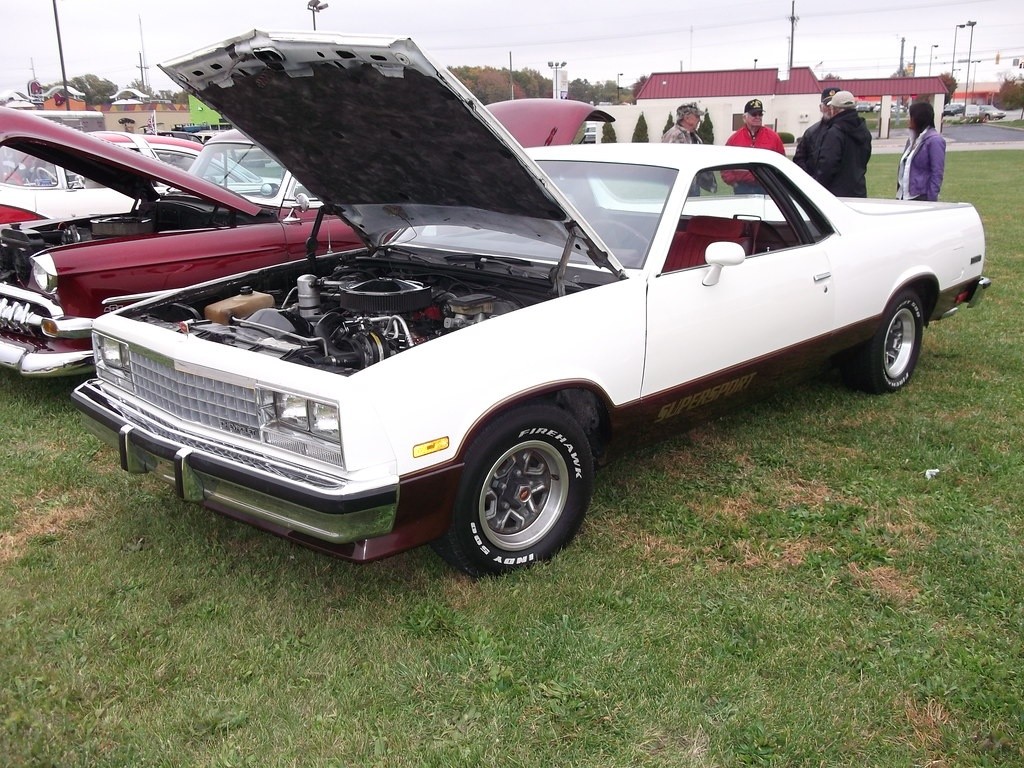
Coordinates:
[548,60,569,100]
[307,0,329,31]
[617,73,625,102]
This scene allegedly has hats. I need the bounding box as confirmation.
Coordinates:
[820,87,841,104]
[677,102,704,120]
[827,91,855,107]
[744,99,766,114]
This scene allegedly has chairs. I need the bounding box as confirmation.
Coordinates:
[660,216,753,272]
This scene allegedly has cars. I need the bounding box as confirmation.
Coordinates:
[71,25,996,580]
[24,107,288,207]
[1,97,616,380]
[854,99,1007,121]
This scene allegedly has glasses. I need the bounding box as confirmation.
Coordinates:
[751,112,763,117]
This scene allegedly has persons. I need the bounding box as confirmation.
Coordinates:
[720,99,785,194]
[895,101,947,202]
[661,102,717,196]
[792,88,872,202]
